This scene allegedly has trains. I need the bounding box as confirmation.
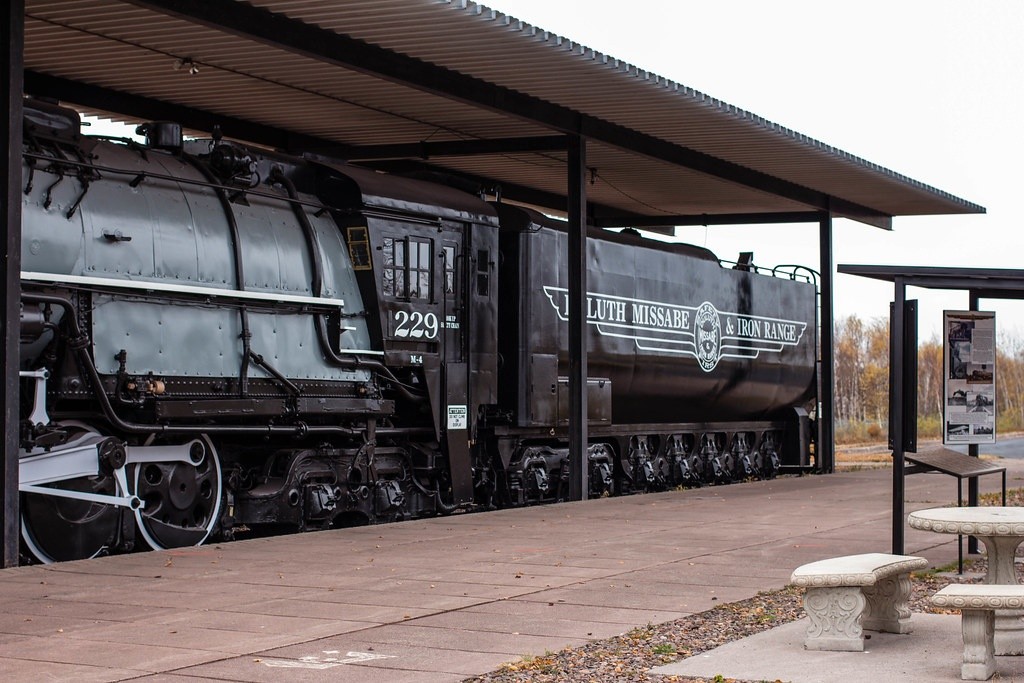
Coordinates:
[18,85,826,565]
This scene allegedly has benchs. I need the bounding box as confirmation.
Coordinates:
[791,553,928,652]
[931,583,1024,681]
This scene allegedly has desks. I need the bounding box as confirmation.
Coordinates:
[907,506,1024,586]
